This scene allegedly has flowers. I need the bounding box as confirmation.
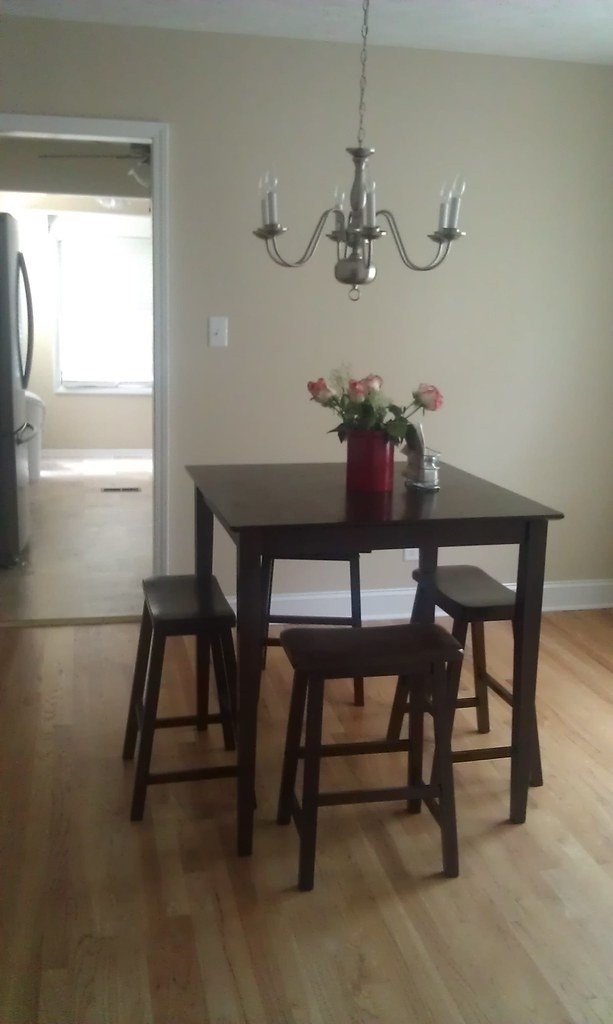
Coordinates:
[308,374,442,445]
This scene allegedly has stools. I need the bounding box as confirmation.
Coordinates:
[277,623,465,893]
[122,575,257,822]
[386,565,542,787]
[261,550,371,707]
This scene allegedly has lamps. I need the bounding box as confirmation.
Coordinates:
[253,0,466,300]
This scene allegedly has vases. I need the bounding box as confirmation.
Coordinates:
[346,430,394,493]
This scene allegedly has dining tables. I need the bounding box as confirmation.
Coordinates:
[185,461,564,856]
